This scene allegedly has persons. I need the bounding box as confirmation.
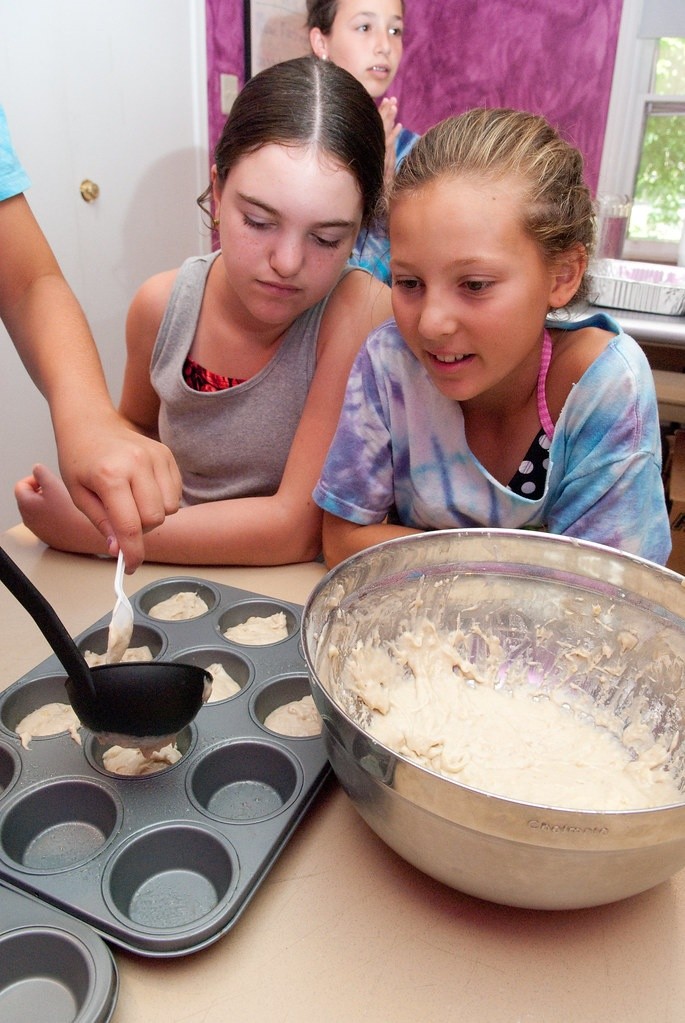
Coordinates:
[298,0,422,289]
[312,108,674,570]
[13,57,394,566]
[0,97,183,575]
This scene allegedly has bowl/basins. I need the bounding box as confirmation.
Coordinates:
[302,527,685,912]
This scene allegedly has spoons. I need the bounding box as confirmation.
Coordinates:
[105,549,134,666]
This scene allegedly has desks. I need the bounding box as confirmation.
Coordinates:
[547,303,685,425]
[0,523,685,1023]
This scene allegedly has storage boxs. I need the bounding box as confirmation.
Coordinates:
[585,257,685,316]
